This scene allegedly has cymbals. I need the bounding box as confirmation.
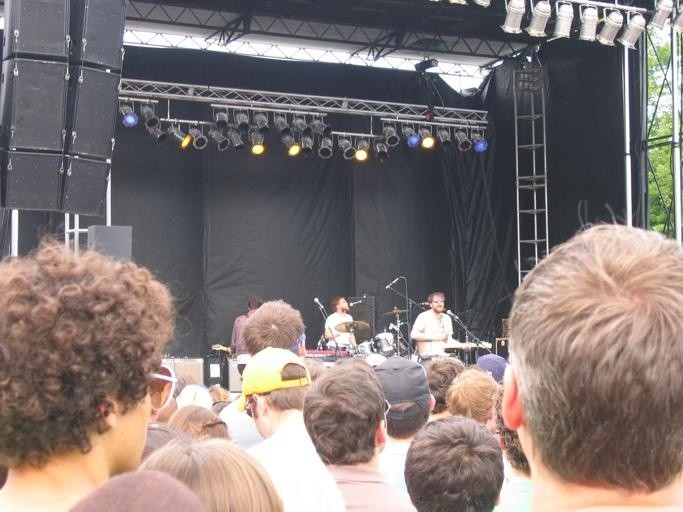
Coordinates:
[382,310,409,316]
[335,321,370,333]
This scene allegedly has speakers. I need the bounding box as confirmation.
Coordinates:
[88,225,132,262]
[0,0,124,219]
[348,295,376,340]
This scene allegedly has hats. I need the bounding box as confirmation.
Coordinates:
[364,353,387,368]
[178,384,212,412]
[374,355,431,421]
[238,346,312,404]
[476,354,508,384]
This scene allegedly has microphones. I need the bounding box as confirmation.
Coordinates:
[350,300,362,306]
[385,277,400,290]
[314,297,324,309]
[447,310,459,320]
[420,302,428,305]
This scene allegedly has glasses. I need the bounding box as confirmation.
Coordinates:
[147,364,176,411]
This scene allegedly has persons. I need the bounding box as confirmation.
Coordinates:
[410,292,471,360]
[70,300,531,511]
[230,296,264,377]
[0,234,207,510]
[325,296,358,349]
[496,224,682,511]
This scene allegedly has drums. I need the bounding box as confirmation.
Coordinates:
[354,354,387,368]
[355,342,375,358]
[320,346,346,351]
[374,332,394,355]
[395,341,406,352]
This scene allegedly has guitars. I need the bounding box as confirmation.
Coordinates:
[417,341,492,360]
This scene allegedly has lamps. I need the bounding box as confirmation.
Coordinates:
[502,0,675,49]
[315,129,388,163]
[119,95,208,150]
[209,103,332,155]
[377,117,489,156]
